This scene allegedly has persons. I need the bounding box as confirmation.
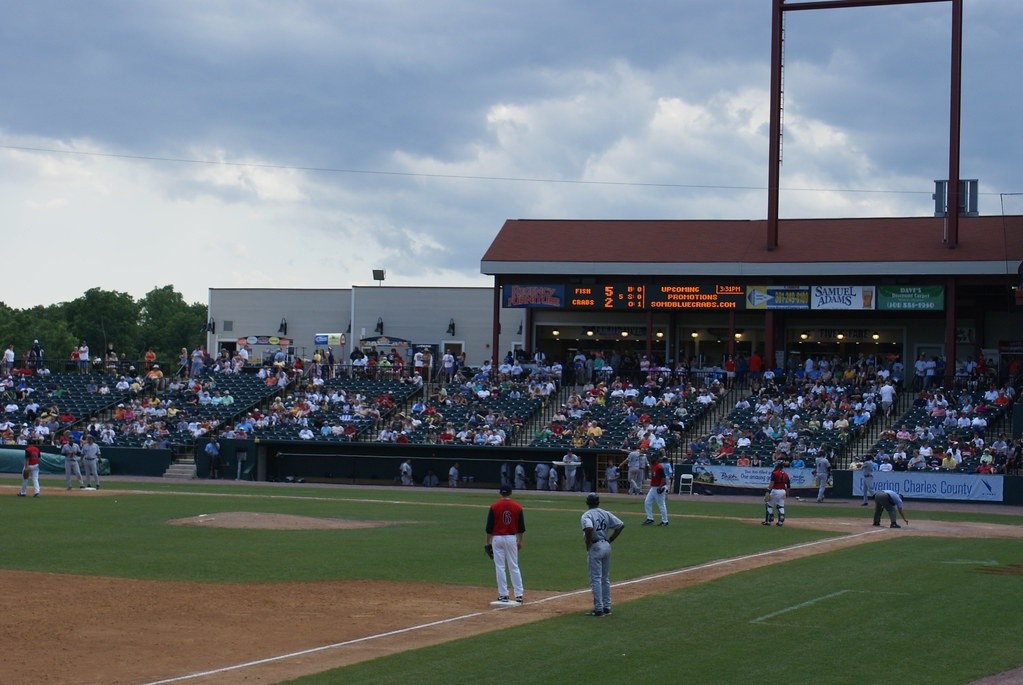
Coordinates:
[61,435,84,490]
[549,465,558,491]
[81,435,102,487]
[449,463,459,487]
[642,454,670,526]
[762,466,790,526]
[605,460,620,493]
[500,461,512,487]
[486,486,526,602]
[535,463,550,489]
[873,490,908,528]
[580,493,624,617]
[856,455,874,506]
[514,458,528,489]
[563,449,578,490]
[18,439,40,497]
[815,451,831,502]
[401,459,412,486]
[0,340,1023,496]
[205,437,220,479]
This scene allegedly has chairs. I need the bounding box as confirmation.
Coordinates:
[0,356,1023,478]
[680,473,693,496]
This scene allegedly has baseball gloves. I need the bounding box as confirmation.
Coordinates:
[657,487,665,494]
[765,492,770,502]
[23,470,29,480]
[856,464,862,468]
[484,544,494,560]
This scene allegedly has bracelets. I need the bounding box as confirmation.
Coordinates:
[609,537,614,541]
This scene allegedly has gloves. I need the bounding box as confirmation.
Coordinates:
[485,545,493,559]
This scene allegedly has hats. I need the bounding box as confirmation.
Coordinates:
[586,492,600,505]
[774,460,784,467]
[657,522,669,528]
[643,519,654,525]
[500,485,512,496]
[649,452,657,457]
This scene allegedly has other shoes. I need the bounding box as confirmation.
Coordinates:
[861,502,868,506]
[95,484,100,487]
[874,522,880,526]
[585,610,603,616]
[516,596,522,602]
[17,492,24,496]
[498,596,509,602]
[80,486,84,489]
[33,494,39,497]
[603,608,612,615]
[890,522,901,529]
[87,484,90,486]
